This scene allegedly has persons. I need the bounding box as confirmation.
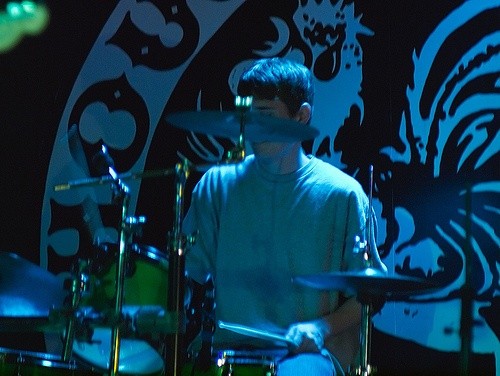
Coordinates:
[95,57,378,376]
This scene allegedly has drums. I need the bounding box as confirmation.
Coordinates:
[61,240,194,376]
[184,350,277,376]
[0,347,79,376]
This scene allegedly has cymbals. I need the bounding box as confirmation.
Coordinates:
[292,268,436,293]
[166,110,318,141]
[1,250,64,318]
